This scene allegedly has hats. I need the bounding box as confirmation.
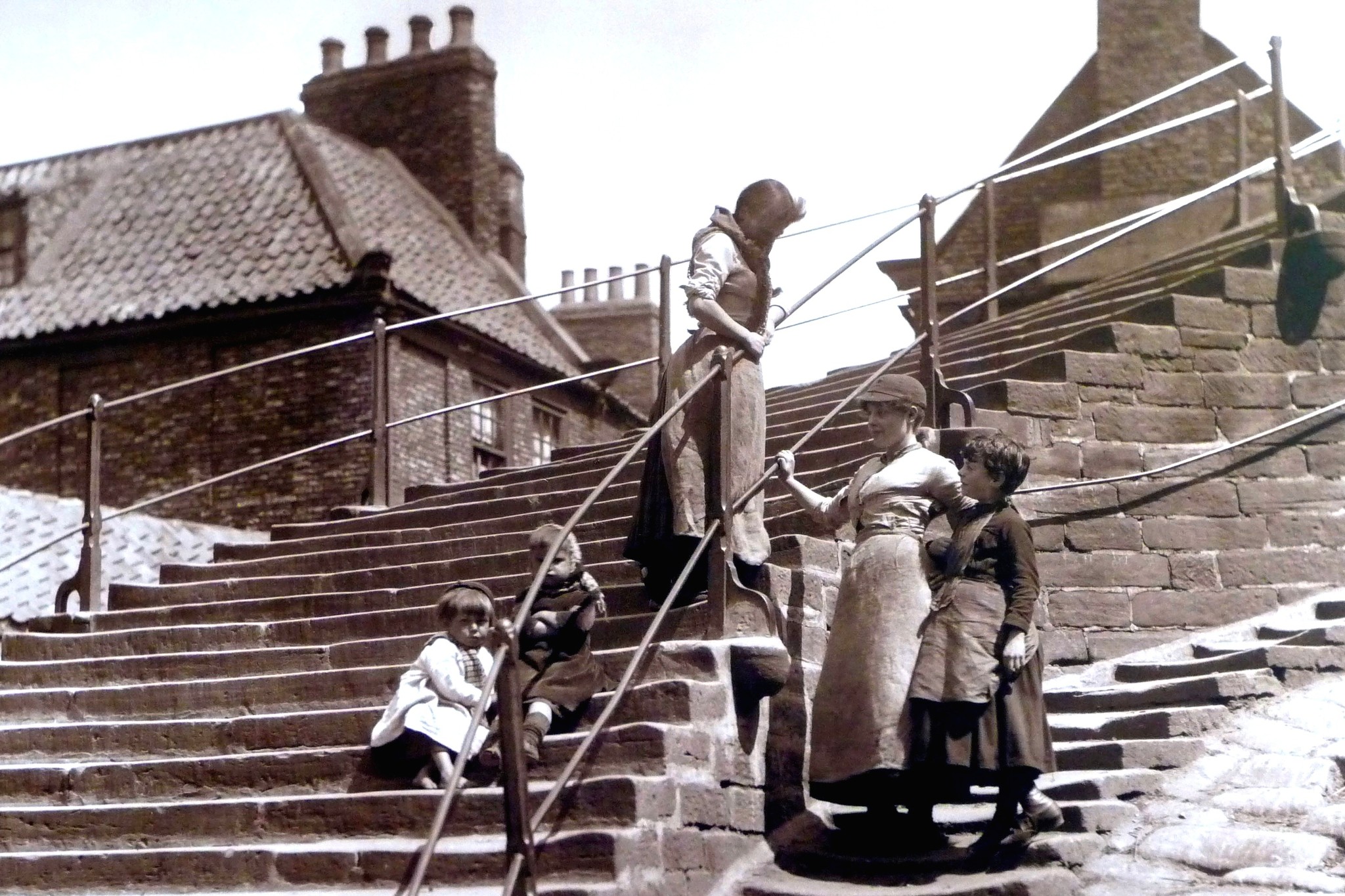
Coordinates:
[857,374,927,409]
[445,580,496,608]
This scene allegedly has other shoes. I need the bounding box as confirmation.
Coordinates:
[481,743,503,767]
[999,801,1064,847]
[524,739,540,762]
[967,815,1017,853]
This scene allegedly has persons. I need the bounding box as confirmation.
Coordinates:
[372,522,602,790]
[773,371,1064,863]
[622,180,805,611]
